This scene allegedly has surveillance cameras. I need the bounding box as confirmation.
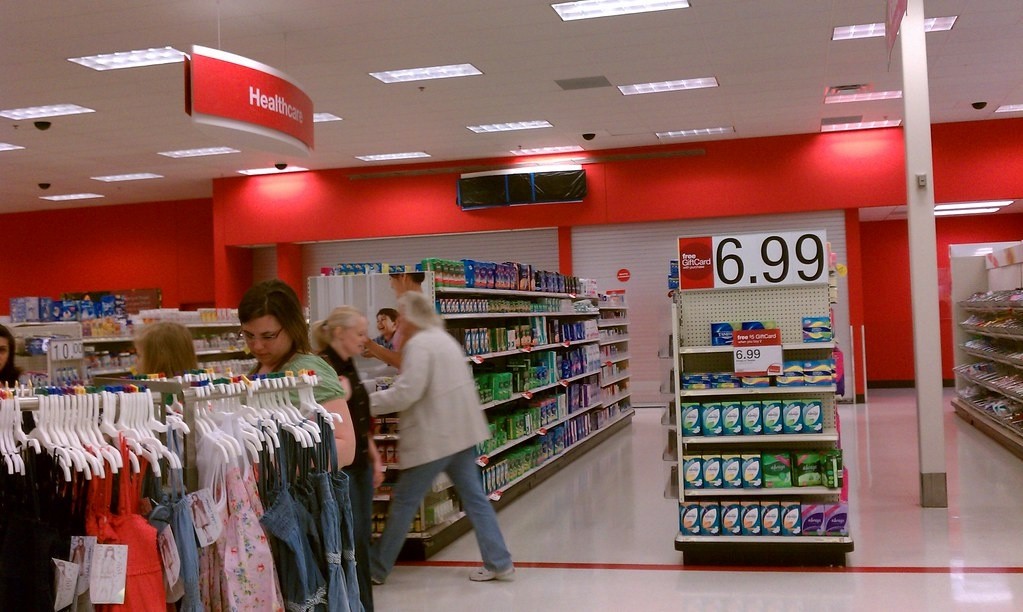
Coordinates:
[34,121,51,131]
[275,163,287,169]
[972,102,987,110]
[39,183,51,189]
[583,133,595,141]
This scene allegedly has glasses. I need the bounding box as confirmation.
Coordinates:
[237,326,283,344]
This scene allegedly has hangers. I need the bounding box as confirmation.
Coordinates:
[0,368,343,482]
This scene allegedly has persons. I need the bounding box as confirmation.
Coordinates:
[0,325,21,388]
[313,306,385,612]
[370,291,516,585]
[234,279,356,471]
[134,321,200,376]
[360,272,426,374]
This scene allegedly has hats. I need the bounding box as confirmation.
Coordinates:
[396,291,438,327]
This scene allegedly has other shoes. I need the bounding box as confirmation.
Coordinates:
[372,577,384,584]
[468,565,515,581]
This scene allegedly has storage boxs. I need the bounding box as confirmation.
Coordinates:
[9,295,127,323]
[421,258,849,539]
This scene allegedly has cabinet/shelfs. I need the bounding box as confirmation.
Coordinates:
[672,301,853,566]
[82,309,259,376]
[950,301,1023,460]
[307,271,635,560]
